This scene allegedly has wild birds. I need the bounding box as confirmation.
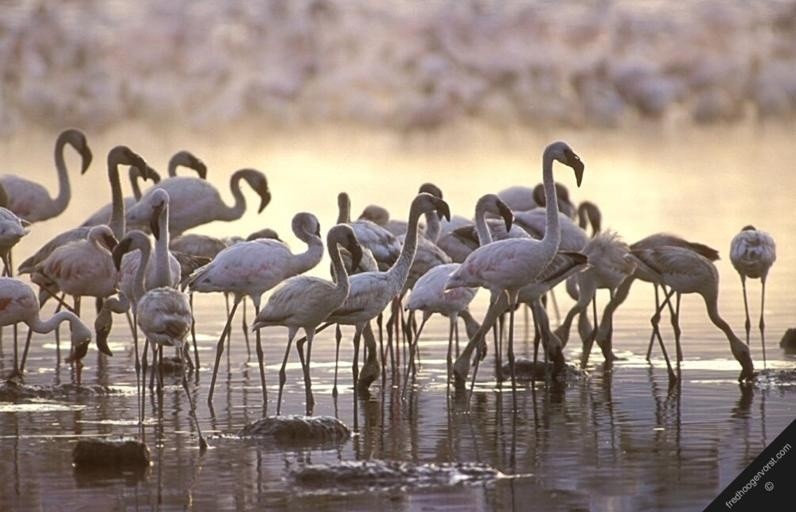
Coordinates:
[0,127,755,416]
[730,224,778,361]
[111,230,208,452]
